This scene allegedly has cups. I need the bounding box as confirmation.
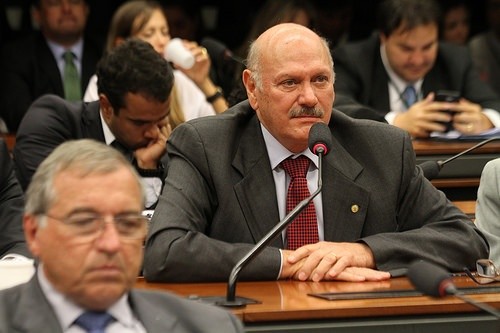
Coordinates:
[164,38,194,69]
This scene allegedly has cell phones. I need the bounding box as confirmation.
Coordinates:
[434,91,460,132]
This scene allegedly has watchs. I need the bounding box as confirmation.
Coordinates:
[206,87,224,104]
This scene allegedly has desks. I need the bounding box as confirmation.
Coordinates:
[411,138,500,188]
[138,271,500,333]
[454,199,476,223]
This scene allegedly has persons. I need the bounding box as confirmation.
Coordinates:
[234,0,318,104]
[474,157,500,267]
[143,22,490,281]
[0,138,39,266]
[331,0,500,139]
[435,0,469,46]
[0,0,105,134]
[0,139,246,333]
[83,0,229,131]
[14,36,175,194]
[159,0,232,90]
[469,0,500,82]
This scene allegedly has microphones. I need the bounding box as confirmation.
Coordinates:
[406,261,500,319]
[190,122,331,309]
[419,137,500,180]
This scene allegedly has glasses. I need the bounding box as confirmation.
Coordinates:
[38,213,148,243]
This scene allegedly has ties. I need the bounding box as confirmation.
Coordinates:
[280,155,319,250]
[75,312,114,333]
[402,87,416,111]
[61,51,81,105]
